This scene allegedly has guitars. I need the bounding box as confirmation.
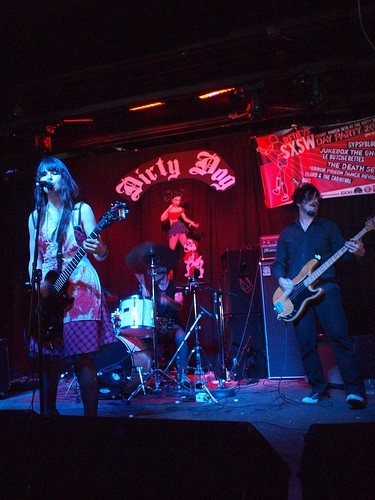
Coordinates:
[271,216,375,322]
[24,200,126,347]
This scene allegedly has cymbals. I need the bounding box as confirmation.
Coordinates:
[125,242,178,276]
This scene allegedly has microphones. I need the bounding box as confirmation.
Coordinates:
[37,180,54,190]
[184,278,191,294]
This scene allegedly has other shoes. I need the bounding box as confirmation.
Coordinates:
[302,391,328,403]
[345,392,366,406]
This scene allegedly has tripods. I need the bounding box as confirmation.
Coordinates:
[126,259,225,406]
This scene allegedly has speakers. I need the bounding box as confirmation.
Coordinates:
[0,335,11,396]
[302,421,375,500]
[0,408,292,500]
[219,247,360,390]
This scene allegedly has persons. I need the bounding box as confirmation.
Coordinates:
[271,183,367,407]
[134,273,189,383]
[28,157,117,417]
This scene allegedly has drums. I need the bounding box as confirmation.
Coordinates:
[117,294,159,338]
[76,334,153,399]
